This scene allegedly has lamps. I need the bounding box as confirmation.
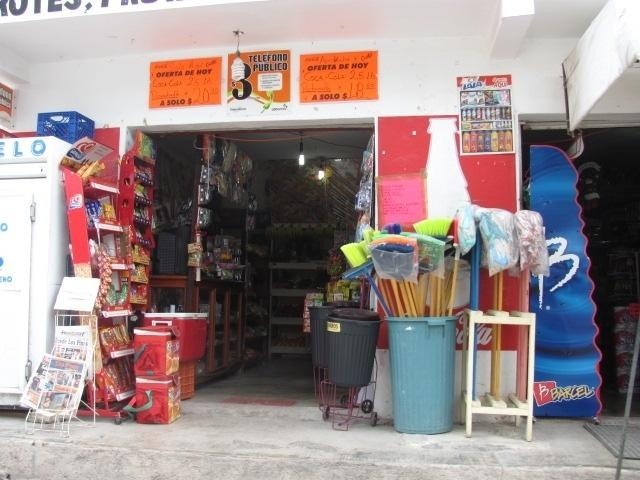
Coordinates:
[298,135,305,166]
[231,30,245,81]
[318,160,325,181]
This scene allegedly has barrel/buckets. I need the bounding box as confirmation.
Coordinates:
[308,306,338,369]
[326,308,381,387]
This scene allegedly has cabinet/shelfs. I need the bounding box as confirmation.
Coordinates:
[268,229,332,361]
[66,153,150,422]
[152,166,246,386]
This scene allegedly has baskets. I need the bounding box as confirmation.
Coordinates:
[36,111,95,145]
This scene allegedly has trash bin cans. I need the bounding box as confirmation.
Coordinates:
[323,308,382,390]
[307,302,326,366]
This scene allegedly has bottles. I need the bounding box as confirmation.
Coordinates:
[461,106,513,151]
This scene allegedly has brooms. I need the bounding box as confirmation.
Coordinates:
[338,213,463,318]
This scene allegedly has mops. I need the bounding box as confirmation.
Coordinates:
[456,202,544,401]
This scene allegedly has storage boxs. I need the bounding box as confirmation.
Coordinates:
[135,378,182,425]
[178,361,195,400]
[133,325,180,381]
[143,312,208,362]
